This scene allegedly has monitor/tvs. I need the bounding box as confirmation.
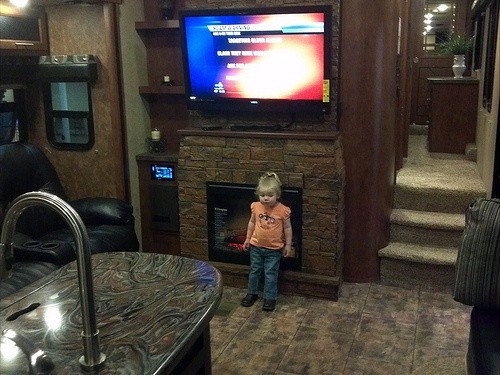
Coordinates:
[179,4,333,114]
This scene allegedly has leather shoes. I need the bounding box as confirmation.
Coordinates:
[262,299,276,310]
[241,293,258,306]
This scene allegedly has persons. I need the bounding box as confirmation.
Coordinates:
[240,172,293,311]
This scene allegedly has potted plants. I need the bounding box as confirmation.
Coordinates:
[438,35,474,79]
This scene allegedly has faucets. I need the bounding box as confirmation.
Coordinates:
[0,190,107,375]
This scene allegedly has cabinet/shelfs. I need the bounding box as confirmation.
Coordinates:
[136,161,182,252]
[429,84,479,153]
[135,20,184,97]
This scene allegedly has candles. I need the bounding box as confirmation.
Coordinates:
[151,127,160,140]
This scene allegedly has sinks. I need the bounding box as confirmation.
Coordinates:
[0,323,54,375]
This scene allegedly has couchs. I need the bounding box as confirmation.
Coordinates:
[0,142,141,299]
